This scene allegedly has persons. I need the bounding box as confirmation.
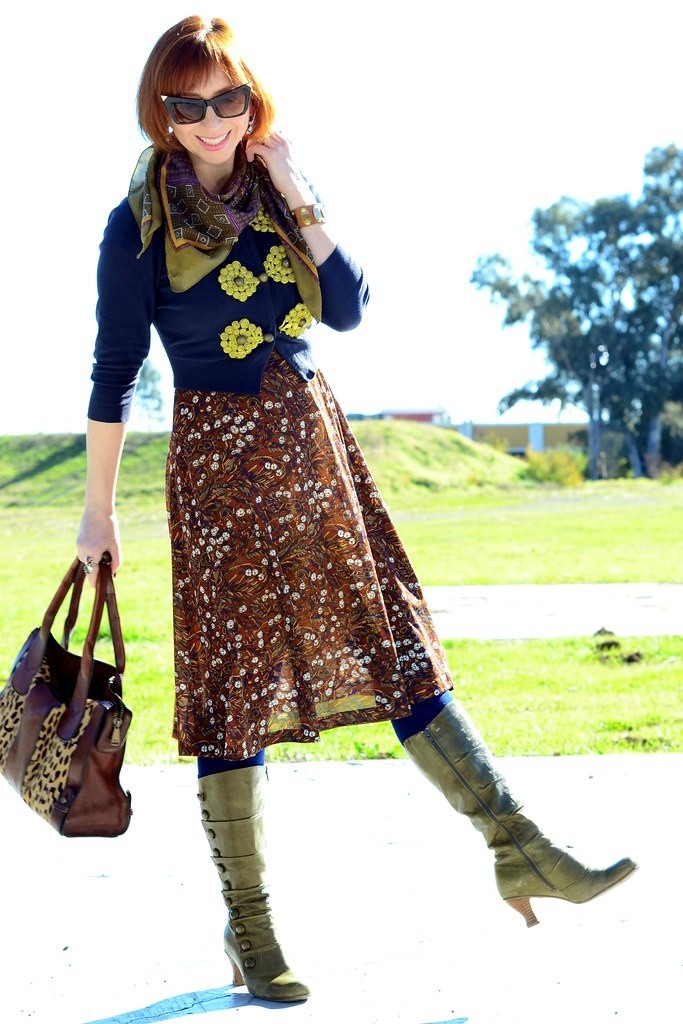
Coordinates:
[1,13,638,1006]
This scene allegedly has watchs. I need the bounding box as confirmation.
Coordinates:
[291,201,330,229]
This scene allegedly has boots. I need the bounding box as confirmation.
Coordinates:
[194,767,314,1005]
[401,701,638,931]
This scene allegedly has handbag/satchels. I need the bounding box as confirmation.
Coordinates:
[0,550,135,841]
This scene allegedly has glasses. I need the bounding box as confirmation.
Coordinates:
[160,81,254,125]
